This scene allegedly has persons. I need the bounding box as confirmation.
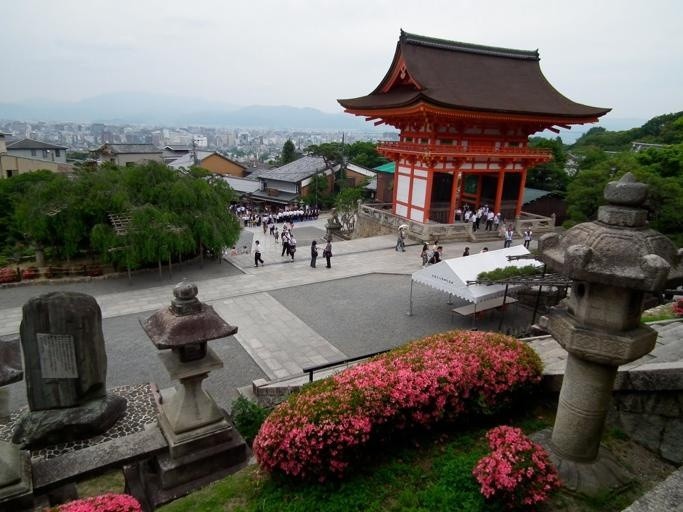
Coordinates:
[462,247,470,256]
[310,240,318,268]
[254,240,264,267]
[396,227,405,251]
[422,239,443,269]
[522,226,533,249]
[455,202,514,249]
[227,201,323,259]
[479,247,488,253]
[401,231,404,248]
[321,240,333,269]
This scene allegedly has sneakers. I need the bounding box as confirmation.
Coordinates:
[472,224,498,234]
[326,265,331,268]
[311,263,315,268]
[393,246,406,252]
[253,261,265,267]
[281,253,294,262]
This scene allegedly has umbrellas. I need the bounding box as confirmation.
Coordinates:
[397,224,408,231]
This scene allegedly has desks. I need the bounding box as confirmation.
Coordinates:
[452,296,518,326]
